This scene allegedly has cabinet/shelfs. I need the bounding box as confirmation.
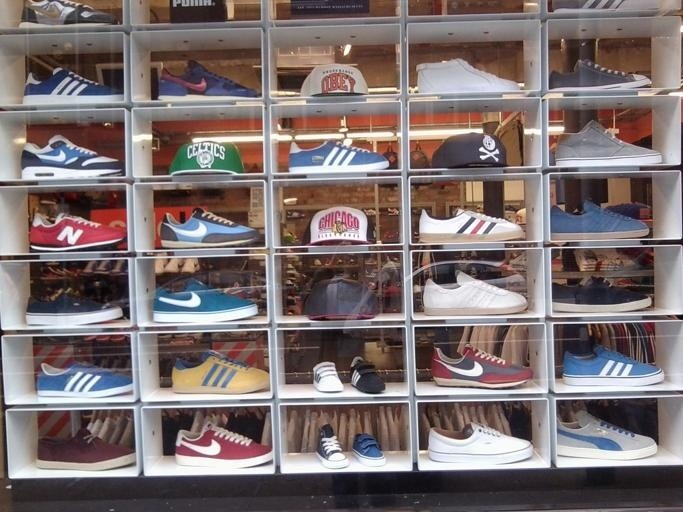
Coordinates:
[0,0,683,498]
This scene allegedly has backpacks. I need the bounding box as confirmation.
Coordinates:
[410,149,431,187]
[377,150,398,187]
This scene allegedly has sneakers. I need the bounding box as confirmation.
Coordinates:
[286,211,306,219]
[310,361,344,393]
[36,362,134,399]
[286,137,392,173]
[347,355,386,393]
[174,420,273,469]
[420,270,529,316]
[18,134,124,179]
[387,208,400,217]
[425,421,534,465]
[561,344,666,386]
[18,66,123,103]
[17,1,119,28]
[171,349,269,396]
[29,210,125,251]
[549,60,652,90]
[160,207,259,249]
[352,433,386,467]
[157,59,263,101]
[550,200,649,238]
[284,256,300,314]
[36,265,78,279]
[314,423,350,471]
[152,277,259,322]
[556,410,658,460]
[34,428,136,472]
[429,341,534,389]
[418,205,525,241]
[79,260,128,276]
[552,276,652,312]
[363,208,382,218]
[24,291,124,326]
[415,58,521,94]
[154,259,200,274]
[552,0,682,17]
[554,119,662,165]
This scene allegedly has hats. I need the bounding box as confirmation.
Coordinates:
[301,206,374,244]
[165,137,246,175]
[300,61,369,97]
[428,132,508,170]
[306,278,377,319]
